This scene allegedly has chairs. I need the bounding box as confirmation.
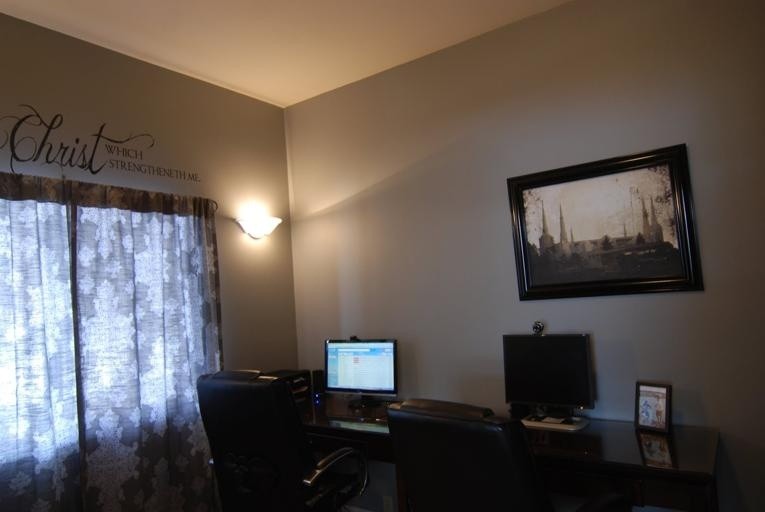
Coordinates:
[197,371,368,512]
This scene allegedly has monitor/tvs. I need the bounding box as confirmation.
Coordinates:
[325,339,398,410]
[503,333,595,433]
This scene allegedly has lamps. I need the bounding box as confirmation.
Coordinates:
[235,216,283,240]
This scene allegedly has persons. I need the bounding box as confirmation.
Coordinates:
[641,401,651,417]
[655,395,663,423]
[644,440,666,460]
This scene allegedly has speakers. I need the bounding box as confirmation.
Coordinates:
[312,368,324,396]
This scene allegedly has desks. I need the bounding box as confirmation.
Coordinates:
[302,388,720,512]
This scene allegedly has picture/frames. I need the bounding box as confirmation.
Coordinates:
[635,383,672,434]
[507,142,704,302]
